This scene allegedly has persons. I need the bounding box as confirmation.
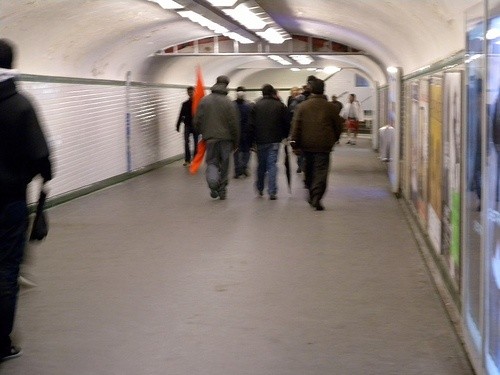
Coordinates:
[0,39,53,362]
[176,75,364,210]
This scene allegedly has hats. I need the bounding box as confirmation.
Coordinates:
[235,86,246,91]
[210,83,229,92]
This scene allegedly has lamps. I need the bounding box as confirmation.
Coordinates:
[151,0,314,65]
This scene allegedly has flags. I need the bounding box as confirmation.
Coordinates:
[189,66,207,175]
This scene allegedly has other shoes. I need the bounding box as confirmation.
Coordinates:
[311,198,323,210]
[209,181,218,198]
[2,344,23,359]
[270,193,277,200]
